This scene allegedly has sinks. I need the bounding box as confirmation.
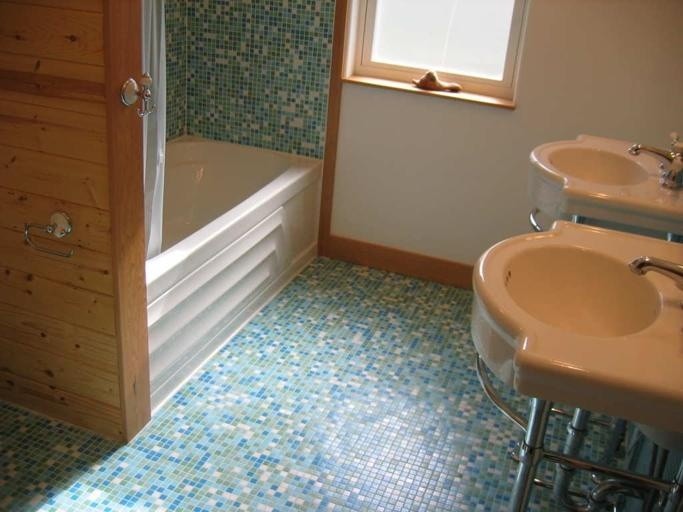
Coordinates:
[471,221,683,450]
[529,135,683,238]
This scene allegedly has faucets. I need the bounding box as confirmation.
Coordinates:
[629,143,676,162]
[629,256,683,290]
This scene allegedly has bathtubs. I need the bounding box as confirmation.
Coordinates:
[144,136,323,419]
